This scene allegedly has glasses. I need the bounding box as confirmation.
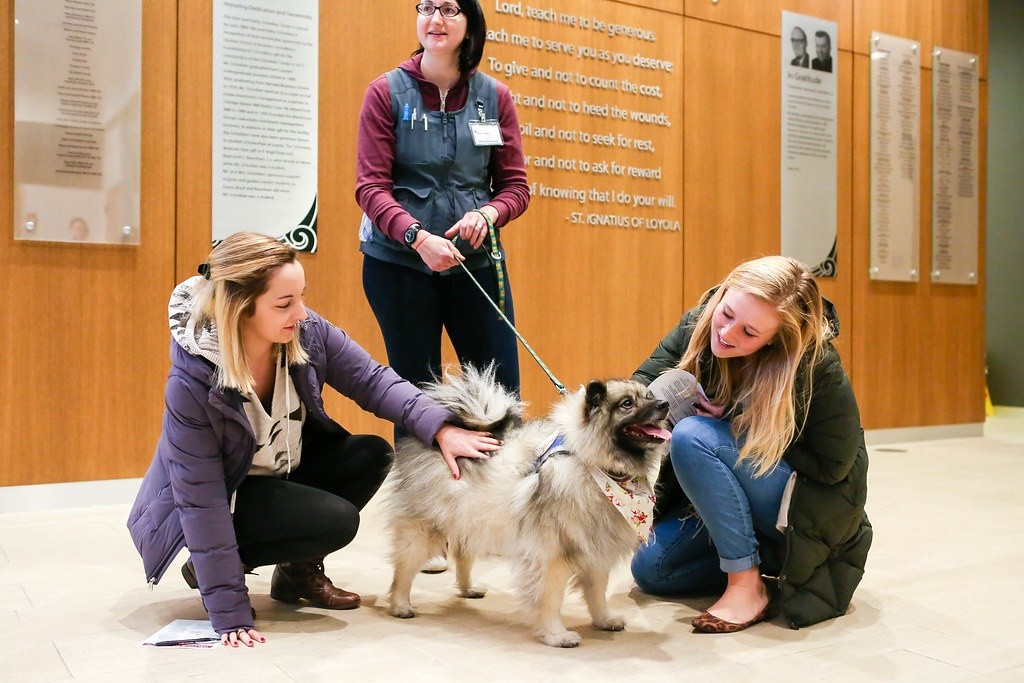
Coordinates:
[792,38,805,43]
[416,3,464,17]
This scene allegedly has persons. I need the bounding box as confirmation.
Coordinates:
[127,232,505,648]
[631,256,873,634]
[788,26,833,73]
[355,0,531,574]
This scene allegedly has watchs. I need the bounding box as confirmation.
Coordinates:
[405,224,423,248]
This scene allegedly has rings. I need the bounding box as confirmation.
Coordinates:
[237,628,244,635]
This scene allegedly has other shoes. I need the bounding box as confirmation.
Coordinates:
[692,591,774,632]
[420,537,449,572]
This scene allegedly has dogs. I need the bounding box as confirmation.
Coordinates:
[383,358,673,648]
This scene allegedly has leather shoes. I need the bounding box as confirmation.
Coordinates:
[181,555,259,620]
[270,559,360,609]
